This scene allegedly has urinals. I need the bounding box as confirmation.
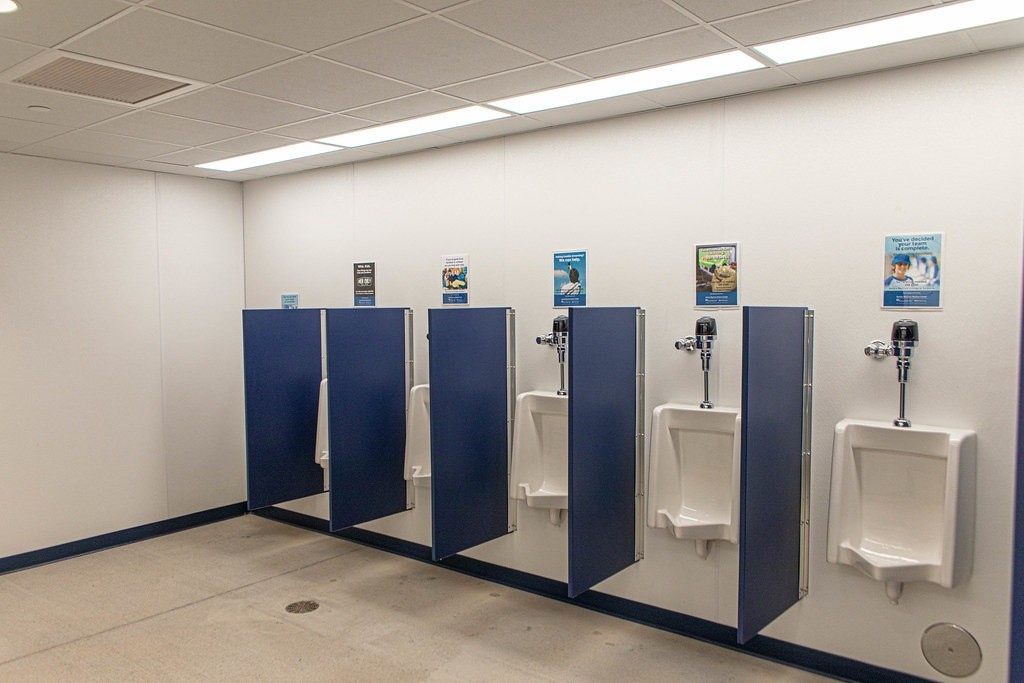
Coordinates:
[646,402,742,544]
[825,418,978,591]
[404,383,430,484]
[314,375,328,466]
[510,390,568,510]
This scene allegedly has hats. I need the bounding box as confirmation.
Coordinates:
[920,256,926,262]
[892,254,911,265]
[931,256,936,261]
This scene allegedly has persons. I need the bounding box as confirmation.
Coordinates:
[559,260,582,295]
[885,254,915,289]
[443,267,467,289]
[928,257,940,285]
[919,257,929,276]
